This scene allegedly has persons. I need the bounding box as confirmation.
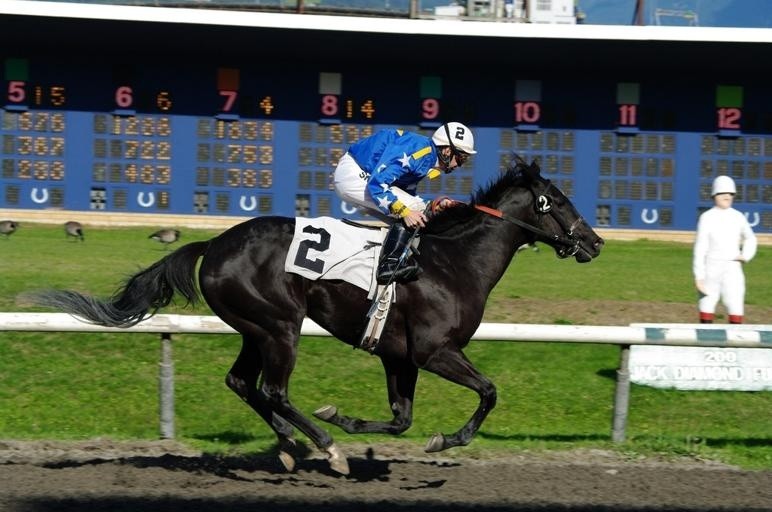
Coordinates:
[693,174,758,324]
[330,122,478,285]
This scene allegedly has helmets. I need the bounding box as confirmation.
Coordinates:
[711,175,736,197]
[432,120,477,155]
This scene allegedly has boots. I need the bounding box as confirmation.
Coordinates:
[376,219,423,283]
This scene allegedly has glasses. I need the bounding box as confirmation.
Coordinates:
[443,123,469,166]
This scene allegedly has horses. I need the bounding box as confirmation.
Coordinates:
[15,151,606,479]
